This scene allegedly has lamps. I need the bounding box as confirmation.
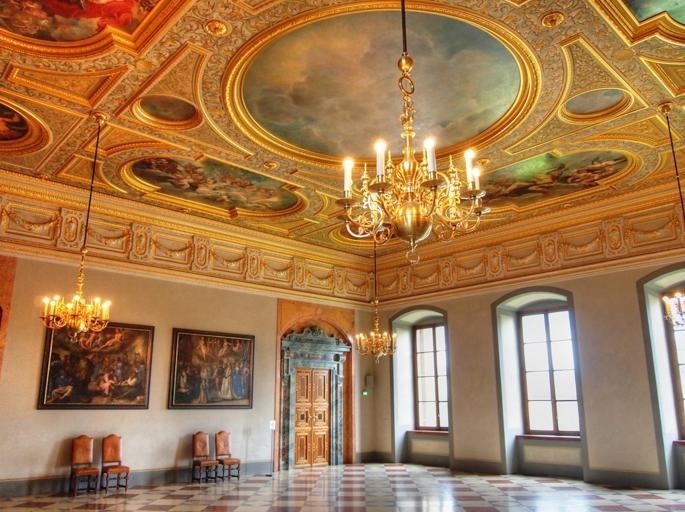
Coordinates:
[339,0,490,264]
[350,237,397,363]
[655,99,685,332]
[37,111,111,335]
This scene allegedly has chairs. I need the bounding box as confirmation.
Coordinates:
[98,434,131,494]
[190,432,219,485]
[213,430,241,484]
[68,433,99,499]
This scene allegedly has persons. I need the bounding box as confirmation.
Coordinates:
[50,348,146,405]
[178,358,248,404]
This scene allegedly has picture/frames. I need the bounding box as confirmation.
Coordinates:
[166,326,256,412]
[36,313,156,412]
